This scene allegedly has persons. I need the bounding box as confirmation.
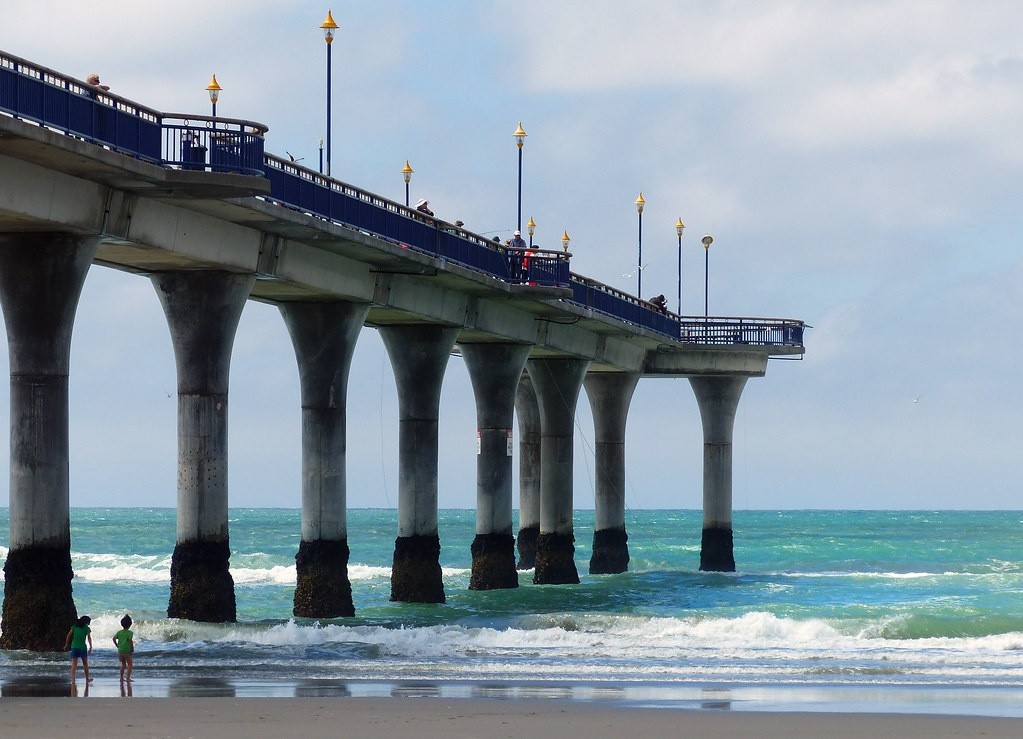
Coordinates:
[64,615,93,685]
[120,682,132,697]
[490,230,551,286]
[415,199,434,225]
[71,685,88,697]
[448,220,464,236]
[113,614,134,682]
[648,294,667,315]
[75,72,110,148]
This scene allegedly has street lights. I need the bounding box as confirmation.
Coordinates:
[701,233,713,344]
[635,192,647,306]
[204,73,223,134]
[513,121,528,239]
[674,216,686,322]
[401,160,415,216]
[527,215,536,248]
[318,7,341,175]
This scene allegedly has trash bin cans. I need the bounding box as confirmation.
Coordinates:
[189,144,205,171]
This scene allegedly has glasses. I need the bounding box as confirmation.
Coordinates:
[94,79,99,82]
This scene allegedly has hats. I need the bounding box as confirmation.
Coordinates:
[454,220,464,226]
[492,235,500,241]
[416,199,428,208]
[514,231,521,236]
